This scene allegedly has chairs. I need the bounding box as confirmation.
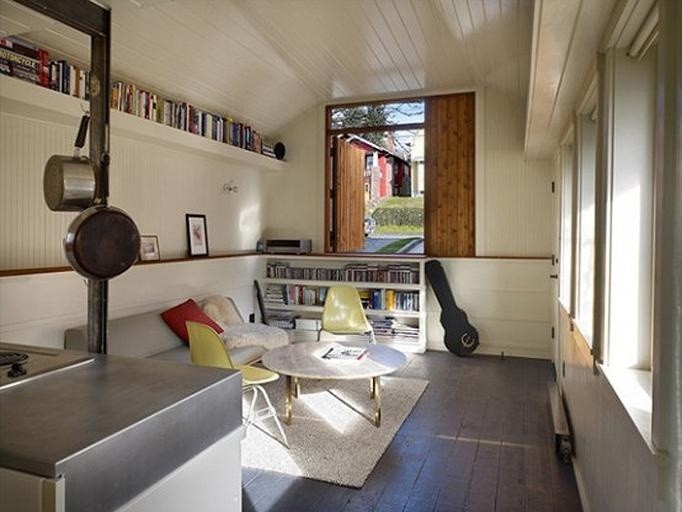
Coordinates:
[185,320,290,449]
[317,285,376,343]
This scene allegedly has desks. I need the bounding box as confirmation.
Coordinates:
[0,341,242,512]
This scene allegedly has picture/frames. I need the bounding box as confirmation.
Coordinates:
[139,236,160,262]
[186,214,209,258]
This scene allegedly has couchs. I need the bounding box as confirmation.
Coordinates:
[64,296,295,365]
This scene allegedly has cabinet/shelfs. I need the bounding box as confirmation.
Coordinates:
[255,255,425,353]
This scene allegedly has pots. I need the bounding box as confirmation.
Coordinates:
[41,109,142,282]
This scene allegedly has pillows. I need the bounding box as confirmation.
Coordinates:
[161,299,223,348]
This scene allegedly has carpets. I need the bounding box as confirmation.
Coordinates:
[242,363,430,489]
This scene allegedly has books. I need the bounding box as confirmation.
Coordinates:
[321,347,369,362]
[262,261,420,344]
[0,35,276,158]
